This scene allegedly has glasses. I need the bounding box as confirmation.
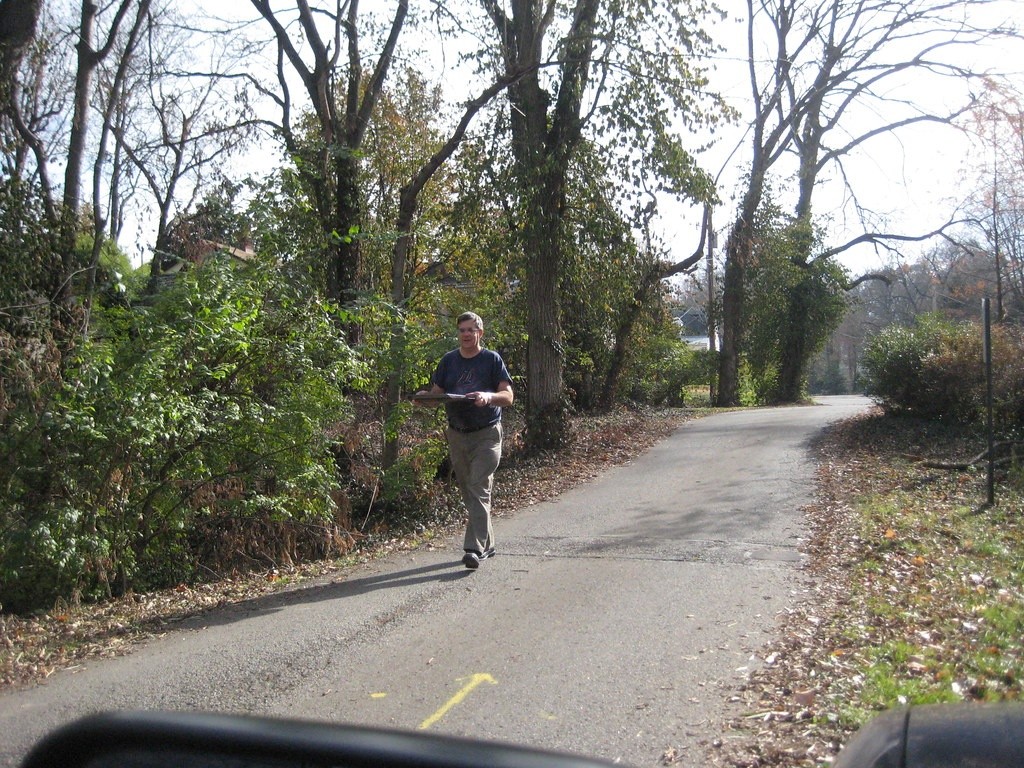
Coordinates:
[459,328,480,332]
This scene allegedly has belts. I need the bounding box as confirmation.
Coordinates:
[449,421,498,433]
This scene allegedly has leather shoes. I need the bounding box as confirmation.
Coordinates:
[479,547,495,561]
[461,552,479,569]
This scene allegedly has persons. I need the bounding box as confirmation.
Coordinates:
[411,312,513,569]
